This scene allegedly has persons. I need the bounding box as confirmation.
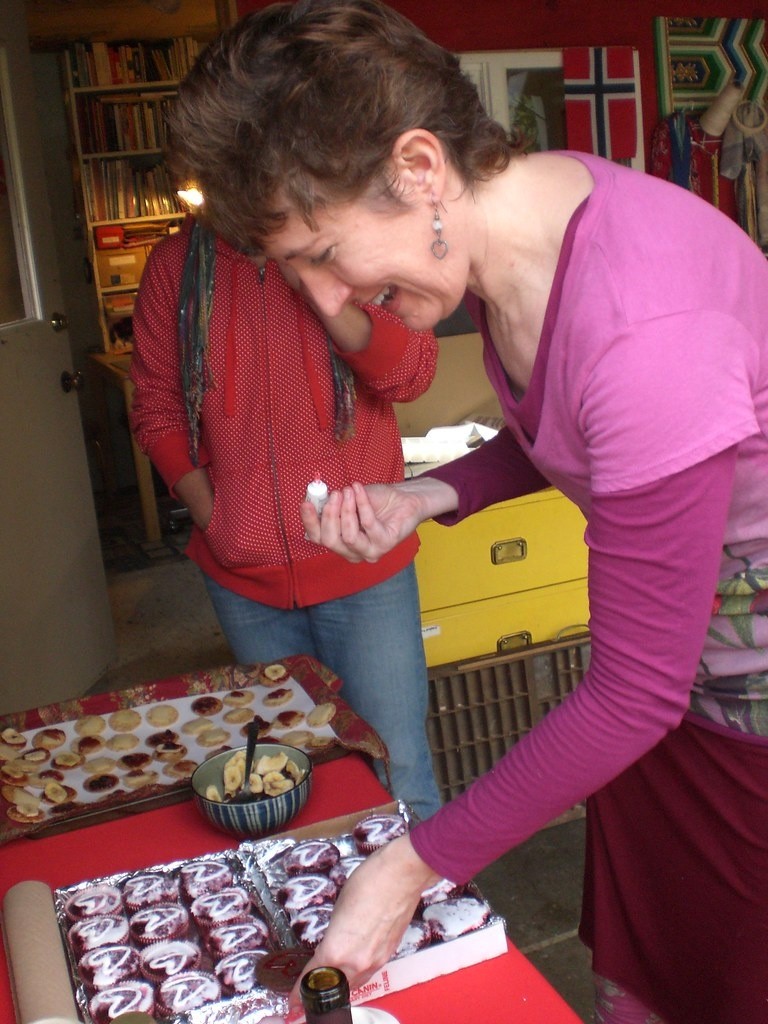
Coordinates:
[167,0,768,1015]
[129,204,443,820]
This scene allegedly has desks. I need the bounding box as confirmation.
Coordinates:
[0,655,588,1024]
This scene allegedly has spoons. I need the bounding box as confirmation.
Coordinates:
[231,722,259,805]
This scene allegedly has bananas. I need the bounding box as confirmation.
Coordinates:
[203,749,305,802]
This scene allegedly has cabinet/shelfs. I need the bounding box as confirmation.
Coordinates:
[54,37,192,570]
[411,487,589,664]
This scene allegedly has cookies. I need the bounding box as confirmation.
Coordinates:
[0,661,232,822]
[222,661,337,750]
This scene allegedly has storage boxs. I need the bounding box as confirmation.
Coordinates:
[95,246,147,286]
[103,292,139,316]
[94,226,125,248]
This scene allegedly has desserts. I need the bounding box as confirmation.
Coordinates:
[64,863,273,1024]
[275,811,490,958]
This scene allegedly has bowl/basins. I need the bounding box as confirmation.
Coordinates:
[190,744,312,834]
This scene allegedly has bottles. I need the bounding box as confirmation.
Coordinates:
[300,966,353,1024]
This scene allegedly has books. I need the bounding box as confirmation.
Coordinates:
[80,158,192,223]
[70,33,210,88]
[122,221,167,250]
[76,92,182,154]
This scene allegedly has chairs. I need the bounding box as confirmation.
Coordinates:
[583,710,768,1024]
